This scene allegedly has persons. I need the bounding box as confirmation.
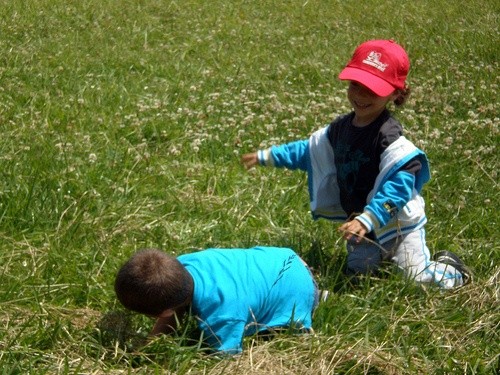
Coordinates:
[241,39,466,290]
[114,245,329,356]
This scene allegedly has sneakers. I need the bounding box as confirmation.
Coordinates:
[434,250,474,285]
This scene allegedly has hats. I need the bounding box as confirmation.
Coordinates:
[338,40,410,97]
